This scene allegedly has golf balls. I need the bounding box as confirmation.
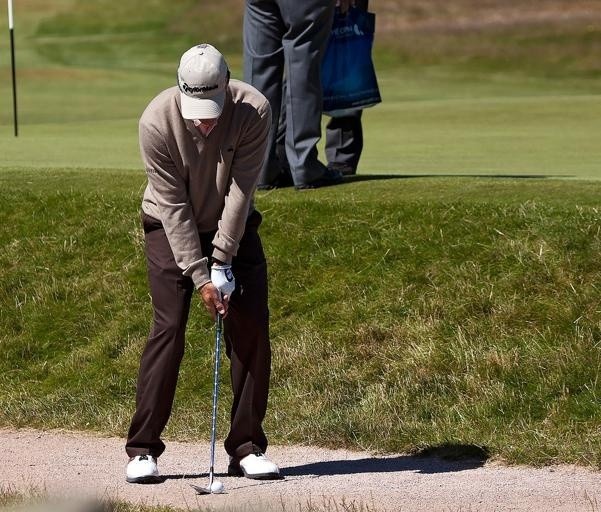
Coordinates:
[211,483,223,494]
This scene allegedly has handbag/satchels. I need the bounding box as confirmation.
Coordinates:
[318,9,383,116]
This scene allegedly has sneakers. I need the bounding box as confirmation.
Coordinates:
[254,151,361,190]
[124,452,161,483]
[225,452,283,482]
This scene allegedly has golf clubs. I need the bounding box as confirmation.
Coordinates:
[189,290,223,493]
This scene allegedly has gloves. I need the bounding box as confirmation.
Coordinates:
[210,263,238,301]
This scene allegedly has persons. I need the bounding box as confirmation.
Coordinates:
[125,43,281,483]
[242,0,369,189]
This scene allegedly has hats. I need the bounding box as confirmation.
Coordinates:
[177,42,228,121]
